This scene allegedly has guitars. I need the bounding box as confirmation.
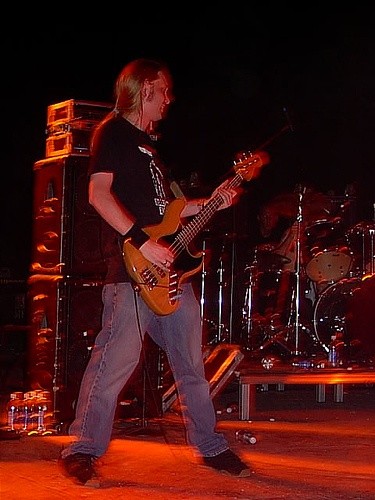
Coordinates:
[122,149,272,316]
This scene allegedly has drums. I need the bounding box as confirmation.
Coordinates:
[346,219,375,279]
[301,220,355,284]
[313,277,361,355]
[249,271,311,359]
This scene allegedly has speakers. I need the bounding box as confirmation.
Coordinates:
[26,154,162,423]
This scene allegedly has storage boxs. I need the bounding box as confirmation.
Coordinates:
[46,99,115,157]
[162,344,245,412]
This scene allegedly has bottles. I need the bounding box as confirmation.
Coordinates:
[4,389,54,435]
[328,333,345,368]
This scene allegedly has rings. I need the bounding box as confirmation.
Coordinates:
[162,261,168,265]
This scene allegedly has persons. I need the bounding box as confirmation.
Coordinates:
[58,60,249,488]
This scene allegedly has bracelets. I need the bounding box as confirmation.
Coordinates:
[122,224,151,249]
[197,200,205,211]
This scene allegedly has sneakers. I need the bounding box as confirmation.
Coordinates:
[197,447,250,478]
[61,452,100,488]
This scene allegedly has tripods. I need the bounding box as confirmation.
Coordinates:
[200,184,329,360]
